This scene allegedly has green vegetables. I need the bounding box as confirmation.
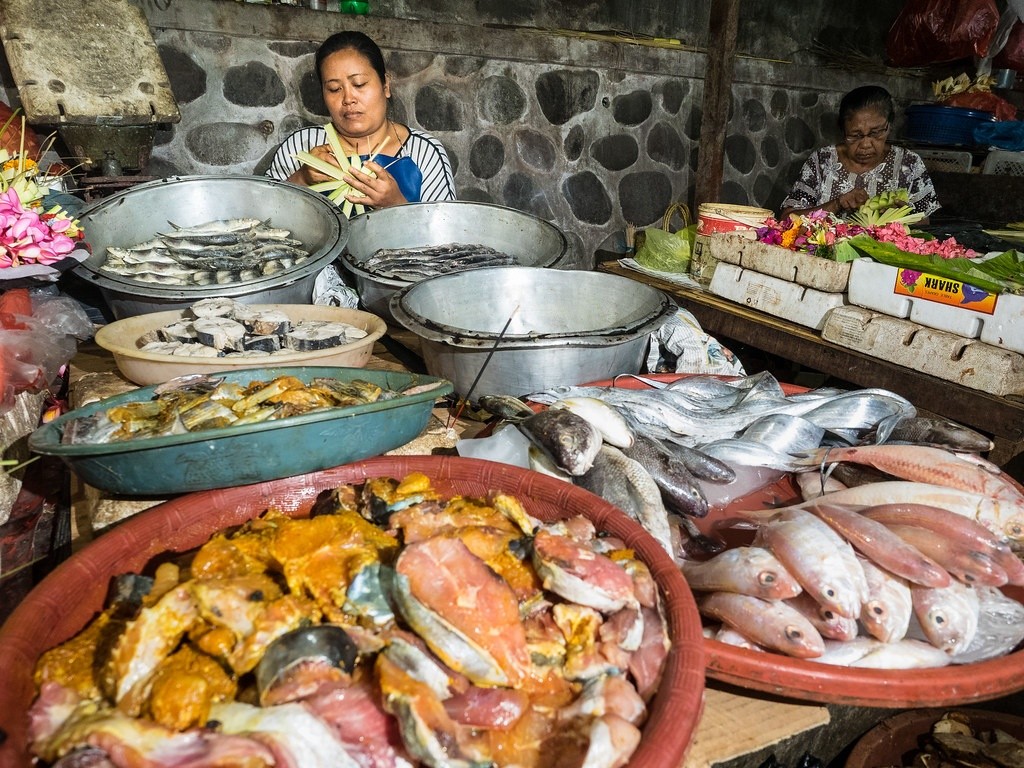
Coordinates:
[634,224,697,273]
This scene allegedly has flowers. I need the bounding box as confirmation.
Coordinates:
[0,107,84,269]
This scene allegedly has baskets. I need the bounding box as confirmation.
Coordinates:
[905,104,1003,147]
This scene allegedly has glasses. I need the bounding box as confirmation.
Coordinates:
[841,120,893,142]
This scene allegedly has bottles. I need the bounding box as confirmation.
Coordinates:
[102,150,123,176]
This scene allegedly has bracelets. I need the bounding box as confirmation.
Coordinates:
[836,198,844,212]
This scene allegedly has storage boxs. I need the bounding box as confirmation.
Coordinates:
[909,147,1024,177]
[0,0,182,126]
[707,232,1024,398]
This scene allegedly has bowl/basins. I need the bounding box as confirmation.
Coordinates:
[338,200,572,328]
[67,174,351,323]
[991,68,1022,90]
[58,125,156,171]
[94,305,386,385]
[37,176,64,192]
[388,266,678,403]
[27,366,453,495]
[0,455,702,768]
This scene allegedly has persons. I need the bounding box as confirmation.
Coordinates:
[261,30,458,222]
[781,86,942,227]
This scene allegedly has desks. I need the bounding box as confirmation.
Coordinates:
[600,261,1023,477]
[70,336,904,768]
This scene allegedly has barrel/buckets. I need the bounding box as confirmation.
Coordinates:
[689,201,774,286]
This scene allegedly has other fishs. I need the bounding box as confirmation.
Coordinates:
[476,369,1024,671]
[135,296,371,360]
[104,215,309,285]
[362,242,524,281]
